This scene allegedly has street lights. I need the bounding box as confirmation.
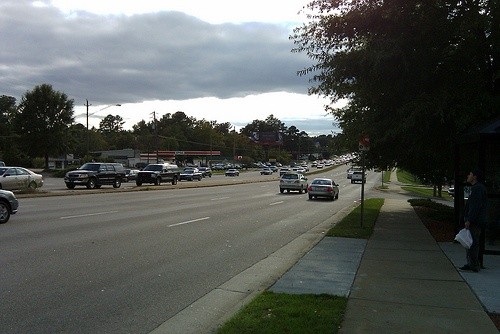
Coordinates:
[86,103,122,132]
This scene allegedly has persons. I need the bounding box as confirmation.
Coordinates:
[459,170,488,272]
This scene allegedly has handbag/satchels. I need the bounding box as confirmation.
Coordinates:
[454,228,473,249]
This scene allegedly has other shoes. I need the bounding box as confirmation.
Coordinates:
[457,264,474,272]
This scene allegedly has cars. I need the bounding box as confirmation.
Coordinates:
[308,178,339,200]
[448,186,473,198]
[124,169,140,182]
[182,167,213,178]
[0,188,20,224]
[225,169,240,176]
[279,172,308,193]
[260,168,273,175]
[177,170,202,182]
[0,167,46,192]
[212,163,268,170]
[347,167,367,184]
[270,166,278,171]
[280,151,360,172]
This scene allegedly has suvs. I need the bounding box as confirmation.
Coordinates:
[135,163,181,187]
[64,163,127,190]
